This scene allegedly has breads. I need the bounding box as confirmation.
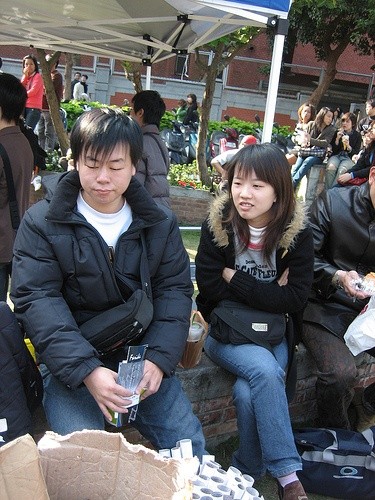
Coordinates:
[364,271,375,286]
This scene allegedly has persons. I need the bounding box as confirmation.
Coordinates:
[288,98,375,207]
[35,53,63,153]
[0,72,35,302]
[71,71,90,102]
[9,106,209,462]
[130,90,172,212]
[195,142,315,500]
[171,94,198,126]
[20,54,44,131]
[307,157,375,434]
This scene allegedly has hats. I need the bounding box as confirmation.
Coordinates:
[242,135,257,147]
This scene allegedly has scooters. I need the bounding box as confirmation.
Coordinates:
[160,108,288,171]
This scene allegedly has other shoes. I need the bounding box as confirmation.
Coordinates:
[31,175,42,192]
[362,387,375,415]
[276,478,308,500]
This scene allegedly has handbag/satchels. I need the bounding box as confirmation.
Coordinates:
[210,299,286,344]
[337,176,369,187]
[299,145,326,157]
[292,427,375,500]
[344,295,375,356]
[78,289,154,359]
